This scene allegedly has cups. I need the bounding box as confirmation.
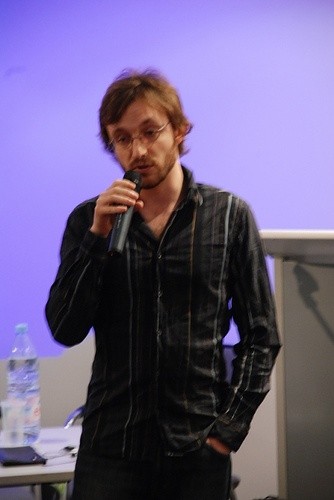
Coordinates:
[0,400,25,446]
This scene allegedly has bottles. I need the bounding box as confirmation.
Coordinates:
[6,324,41,445]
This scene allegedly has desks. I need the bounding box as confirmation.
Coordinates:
[0,426,83,500]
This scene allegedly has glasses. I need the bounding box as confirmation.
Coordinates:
[107,120,171,150]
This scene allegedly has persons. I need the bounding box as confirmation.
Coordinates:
[44,68,283,500]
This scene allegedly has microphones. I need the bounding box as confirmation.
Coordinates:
[107,170,142,256]
